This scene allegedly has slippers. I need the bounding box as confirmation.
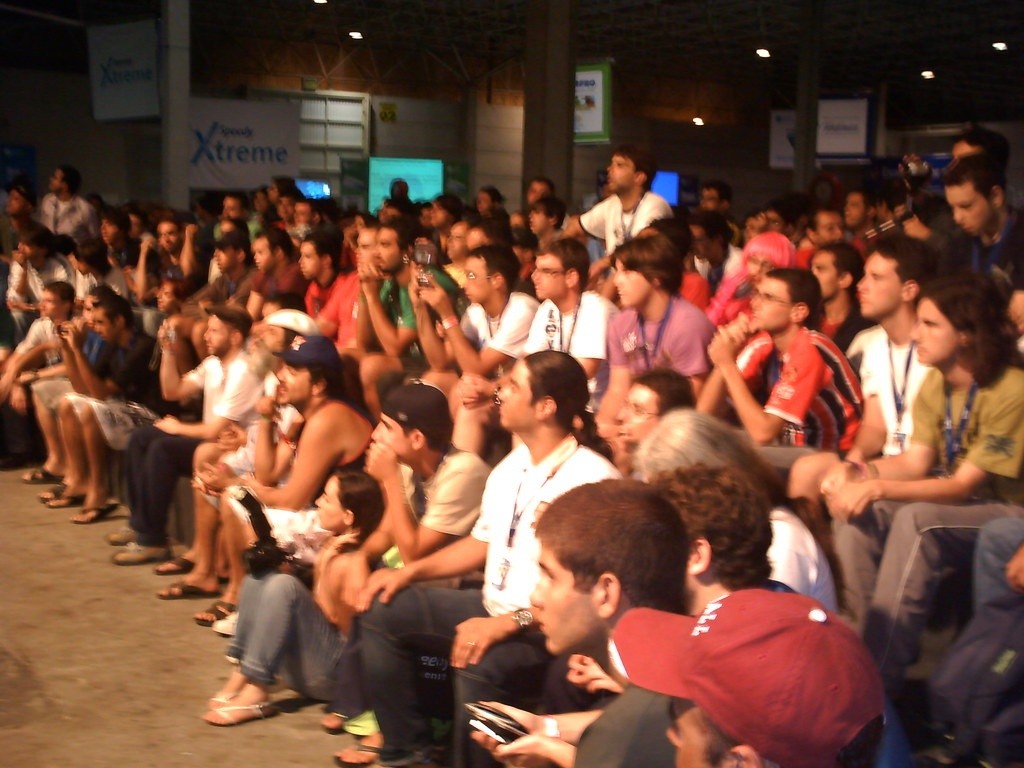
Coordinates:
[159,581,221,600]
[48,493,86,509]
[41,482,68,503]
[335,740,383,767]
[23,467,63,483]
[155,556,195,575]
[197,600,237,625]
[76,501,118,524]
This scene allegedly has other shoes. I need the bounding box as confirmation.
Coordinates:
[113,542,168,564]
[108,527,138,545]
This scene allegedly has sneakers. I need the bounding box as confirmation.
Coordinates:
[213,612,239,634]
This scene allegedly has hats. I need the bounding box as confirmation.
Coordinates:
[204,306,253,337]
[272,333,342,372]
[382,379,457,438]
[608,589,885,768]
[268,307,320,337]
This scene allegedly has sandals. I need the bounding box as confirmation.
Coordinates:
[209,697,229,709]
[205,699,277,726]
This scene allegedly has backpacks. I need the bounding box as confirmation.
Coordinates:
[923,592,1024,762]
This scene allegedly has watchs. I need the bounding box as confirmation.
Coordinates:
[513,607,533,627]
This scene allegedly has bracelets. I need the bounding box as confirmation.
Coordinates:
[866,463,879,480]
[899,211,914,222]
[545,717,558,738]
[442,316,457,329]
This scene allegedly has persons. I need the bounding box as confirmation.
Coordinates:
[0,127,1024,768]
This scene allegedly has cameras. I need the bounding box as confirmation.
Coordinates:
[226,484,287,578]
[417,273,433,287]
[166,330,177,343]
[57,324,69,336]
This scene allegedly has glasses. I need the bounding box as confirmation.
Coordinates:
[749,290,796,308]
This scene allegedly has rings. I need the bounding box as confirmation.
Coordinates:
[466,642,476,645]
